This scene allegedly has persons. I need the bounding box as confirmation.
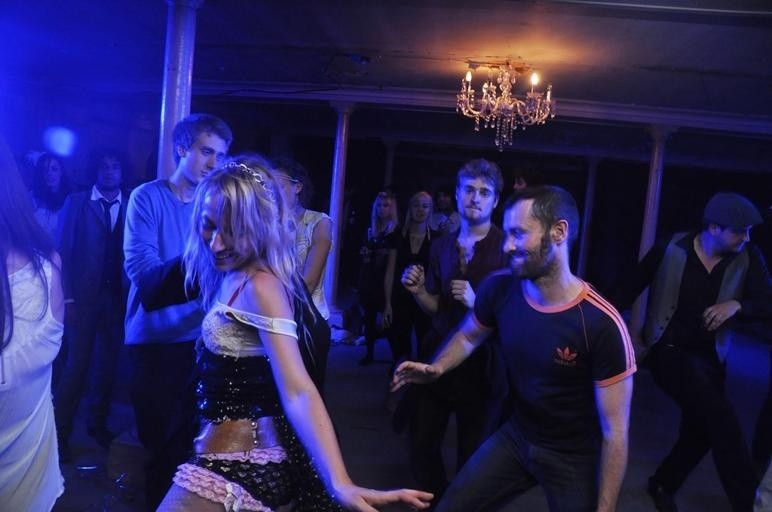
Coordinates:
[2,134,65,511]
[29,150,75,253]
[341,207,365,343]
[385,191,430,392]
[123,113,236,512]
[342,192,399,364]
[156,156,434,511]
[600,193,756,511]
[430,187,460,235]
[508,173,529,197]
[390,186,636,512]
[401,163,507,494]
[52,153,128,438]
[268,159,329,328]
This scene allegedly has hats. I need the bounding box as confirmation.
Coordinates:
[706,193,762,228]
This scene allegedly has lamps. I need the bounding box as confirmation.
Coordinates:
[456,58,555,152]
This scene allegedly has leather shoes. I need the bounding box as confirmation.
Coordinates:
[649,474,677,512]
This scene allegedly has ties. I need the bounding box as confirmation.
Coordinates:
[102,199,118,231]
